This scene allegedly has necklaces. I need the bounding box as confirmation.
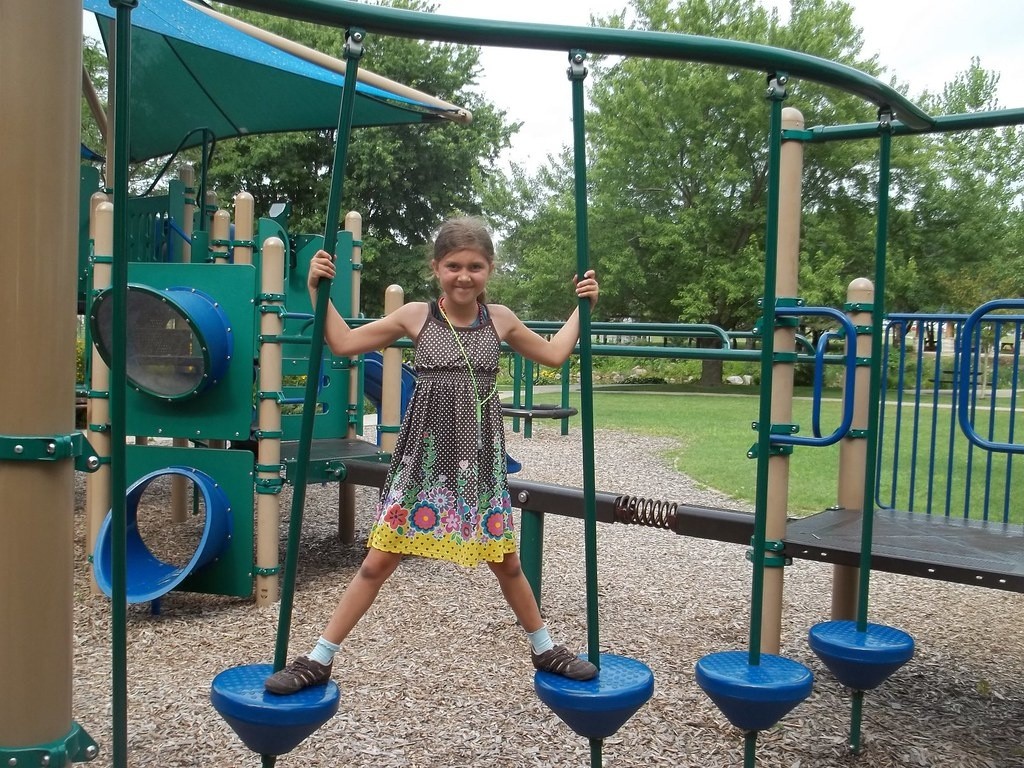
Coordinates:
[439,296,482,325]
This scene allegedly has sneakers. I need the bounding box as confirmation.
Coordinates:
[531,644,598,681]
[265,655,334,695]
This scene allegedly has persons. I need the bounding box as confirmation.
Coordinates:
[263,219,598,696]
